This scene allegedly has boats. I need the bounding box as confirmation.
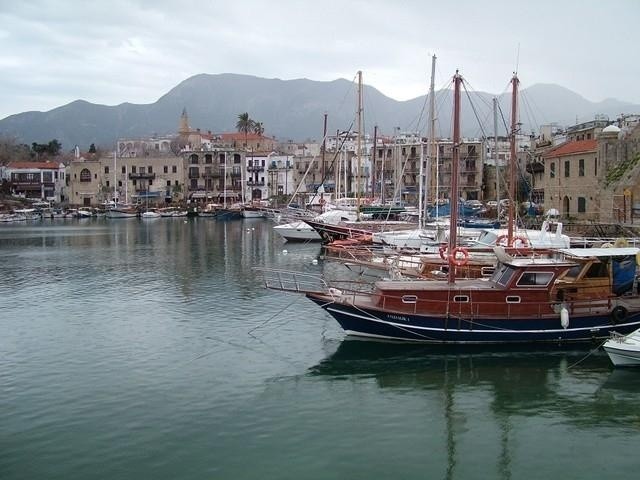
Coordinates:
[602,327,640,368]
[0,202,281,222]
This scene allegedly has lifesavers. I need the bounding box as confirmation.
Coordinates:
[496,235,509,245]
[508,236,529,256]
[440,243,450,261]
[450,247,469,267]
